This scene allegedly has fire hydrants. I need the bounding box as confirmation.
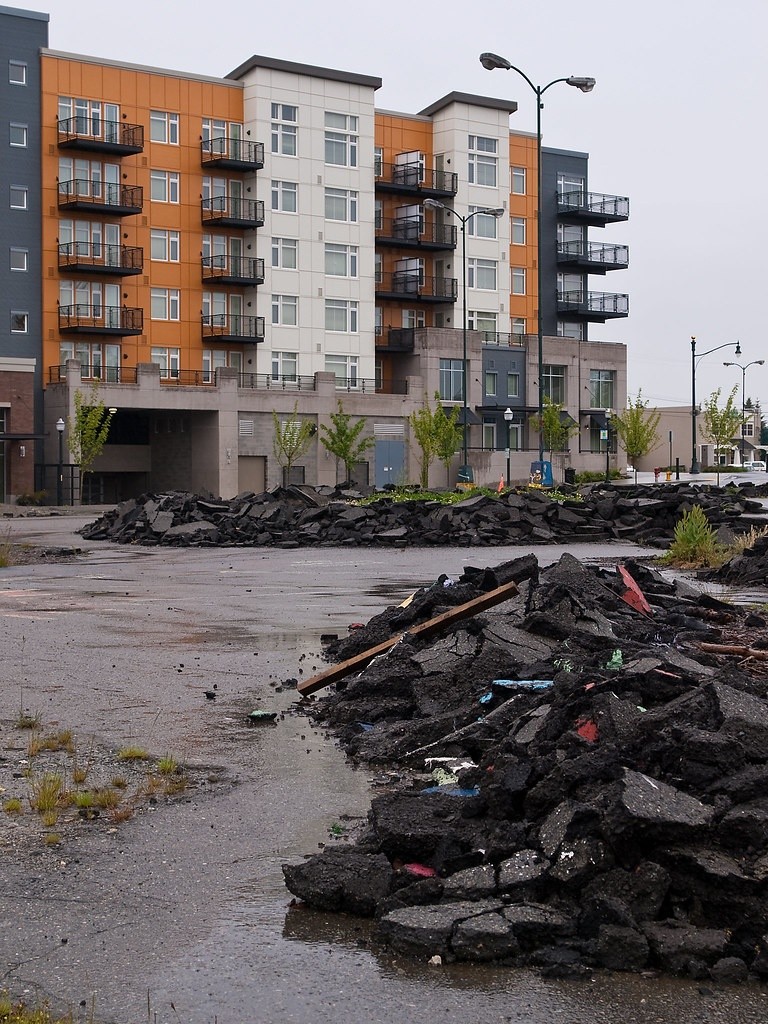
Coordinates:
[654,466,660,482]
[666,471,672,480]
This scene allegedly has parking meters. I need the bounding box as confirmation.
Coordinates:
[722,359,767,468]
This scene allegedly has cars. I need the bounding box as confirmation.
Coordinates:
[742,461,767,471]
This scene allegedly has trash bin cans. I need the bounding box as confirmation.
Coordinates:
[564,466,576,484]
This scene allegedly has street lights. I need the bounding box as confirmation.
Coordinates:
[419,198,507,466]
[690,335,742,475]
[476,50,597,491]
[57,418,66,506]
[604,408,612,483]
[503,408,515,487]
